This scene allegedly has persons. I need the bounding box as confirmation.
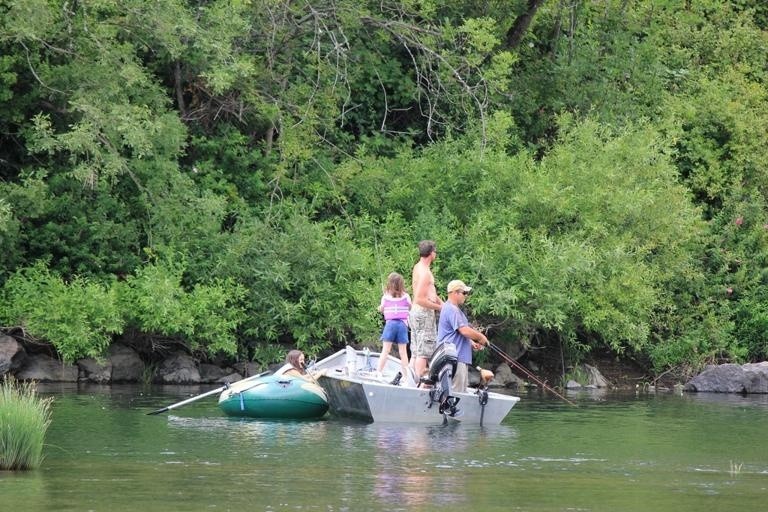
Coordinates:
[468,339,495,383]
[273,349,330,383]
[435,279,490,393]
[408,239,445,390]
[376,271,412,375]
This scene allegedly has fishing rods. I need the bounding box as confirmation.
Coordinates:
[472,341,577,408]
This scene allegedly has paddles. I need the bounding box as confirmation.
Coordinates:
[147,370,269,415]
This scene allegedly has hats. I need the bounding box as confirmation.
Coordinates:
[446,279,473,292]
